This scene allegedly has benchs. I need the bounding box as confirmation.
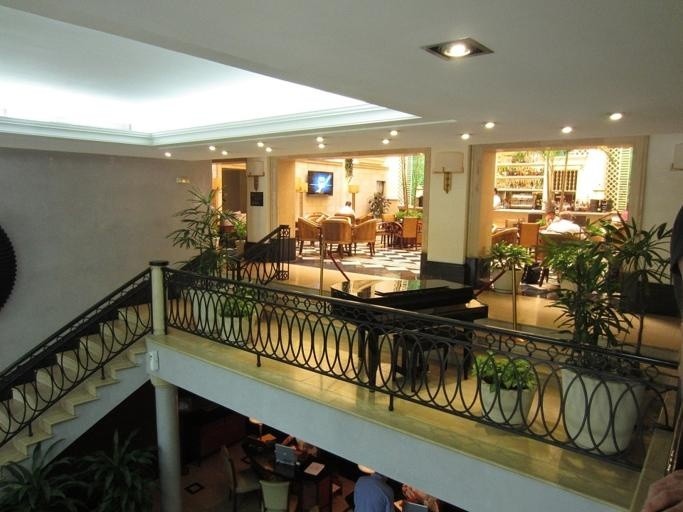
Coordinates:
[393,326,469,392]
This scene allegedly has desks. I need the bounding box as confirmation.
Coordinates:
[345,490,354,510]
[242,438,332,511]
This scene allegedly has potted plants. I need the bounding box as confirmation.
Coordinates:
[217,296,255,347]
[479,240,535,294]
[531,209,682,456]
[471,349,539,428]
[368,192,391,233]
[233,221,247,257]
[167,186,245,328]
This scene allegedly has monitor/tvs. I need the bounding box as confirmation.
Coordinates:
[307,171,333,196]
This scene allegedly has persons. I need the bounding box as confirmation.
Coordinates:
[546,210,584,233]
[641,468,683,512]
[503,199,509,209]
[669,204,683,324]
[338,201,354,216]
[353,472,440,512]
[544,211,560,230]
[282,435,330,512]
[493,189,501,209]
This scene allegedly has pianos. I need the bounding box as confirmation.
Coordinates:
[330,278,488,393]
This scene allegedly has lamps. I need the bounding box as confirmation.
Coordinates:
[432,151,464,193]
[246,160,265,189]
[670,143,683,170]
[248,417,264,437]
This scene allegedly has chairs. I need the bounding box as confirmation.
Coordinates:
[490,215,596,286]
[293,213,423,257]
[258,480,290,512]
[223,446,261,512]
